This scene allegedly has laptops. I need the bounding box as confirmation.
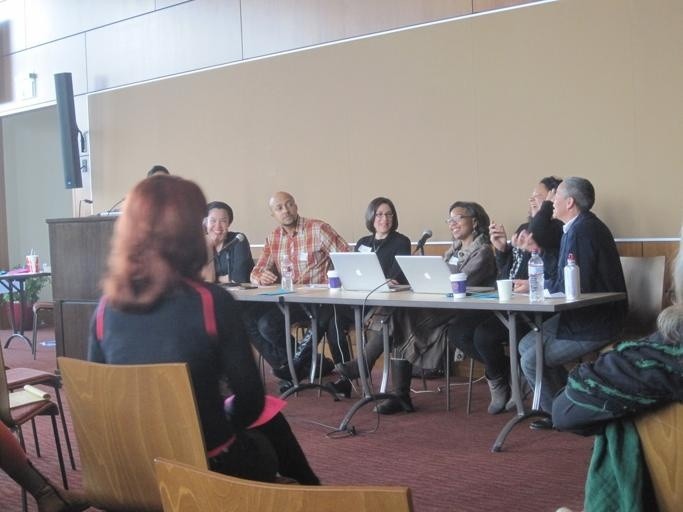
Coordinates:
[395,254,496,295]
[329,252,410,292]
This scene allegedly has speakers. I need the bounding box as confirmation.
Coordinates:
[54,73,83,191]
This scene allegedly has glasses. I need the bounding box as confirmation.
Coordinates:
[446,214,473,224]
[376,213,393,218]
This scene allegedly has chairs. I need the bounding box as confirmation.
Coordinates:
[0,348,74,512]
[621,254,667,336]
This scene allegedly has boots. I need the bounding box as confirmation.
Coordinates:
[484,355,532,415]
[334,332,383,379]
[326,341,351,398]
[8,457,90,511]
[372,358,413,414]
[273,327,323,383]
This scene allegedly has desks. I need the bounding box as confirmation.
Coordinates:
[0,267,50,354]
[207,279,628,453]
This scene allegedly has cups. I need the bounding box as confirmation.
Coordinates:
[25,255,39,274]
[496,280,512,303]
[328,270,342,290]
[449,273,467,299]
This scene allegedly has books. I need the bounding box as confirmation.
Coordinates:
[8,383,50,409]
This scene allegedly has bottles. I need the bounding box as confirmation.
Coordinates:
[564,253,581,299]
[281,255,294,290]
[527,248,545,300]
[42,263,49,272]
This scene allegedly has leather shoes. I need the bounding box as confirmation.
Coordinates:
[529,417,552,429]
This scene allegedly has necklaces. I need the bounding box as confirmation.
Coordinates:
[373,244,379,253]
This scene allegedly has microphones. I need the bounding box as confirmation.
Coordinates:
[215,232,245,256]
[78,199,94,218]
[412,230,432,254]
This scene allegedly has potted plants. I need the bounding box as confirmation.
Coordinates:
[2,267,51,331]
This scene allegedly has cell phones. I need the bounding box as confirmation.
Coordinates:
[241,286,258,289]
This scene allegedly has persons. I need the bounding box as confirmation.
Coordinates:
[146,164,170,178]
[241,190,350,394]
[200,200,255,285]
[548,247,682,437]
[508,176,630,431]
[444,176,562,415]
[333,200,495,415]
[270,196,412,399]
[83,174,320,485]
